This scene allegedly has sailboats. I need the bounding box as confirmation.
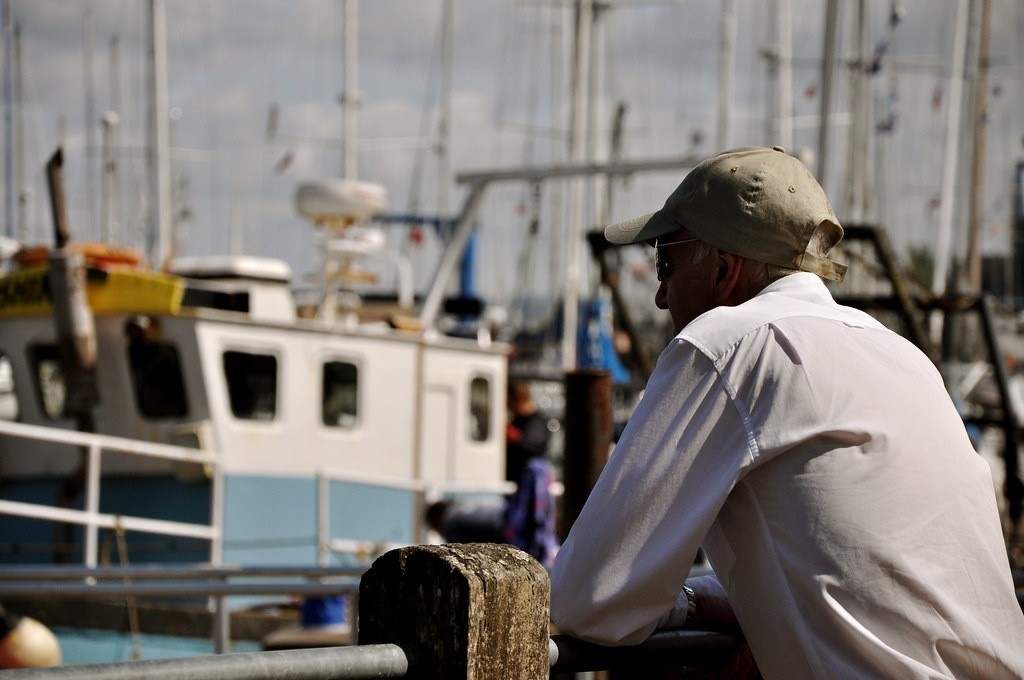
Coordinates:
[1,1,1024,638]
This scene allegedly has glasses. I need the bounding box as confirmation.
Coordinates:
[654,237,698,272]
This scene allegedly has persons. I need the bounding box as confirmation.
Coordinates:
[551,146,1024,680]
[507,381,556,560]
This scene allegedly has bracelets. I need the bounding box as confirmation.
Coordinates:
[682,585,695,622]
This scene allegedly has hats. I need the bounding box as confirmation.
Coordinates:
[604,145,849,283]
